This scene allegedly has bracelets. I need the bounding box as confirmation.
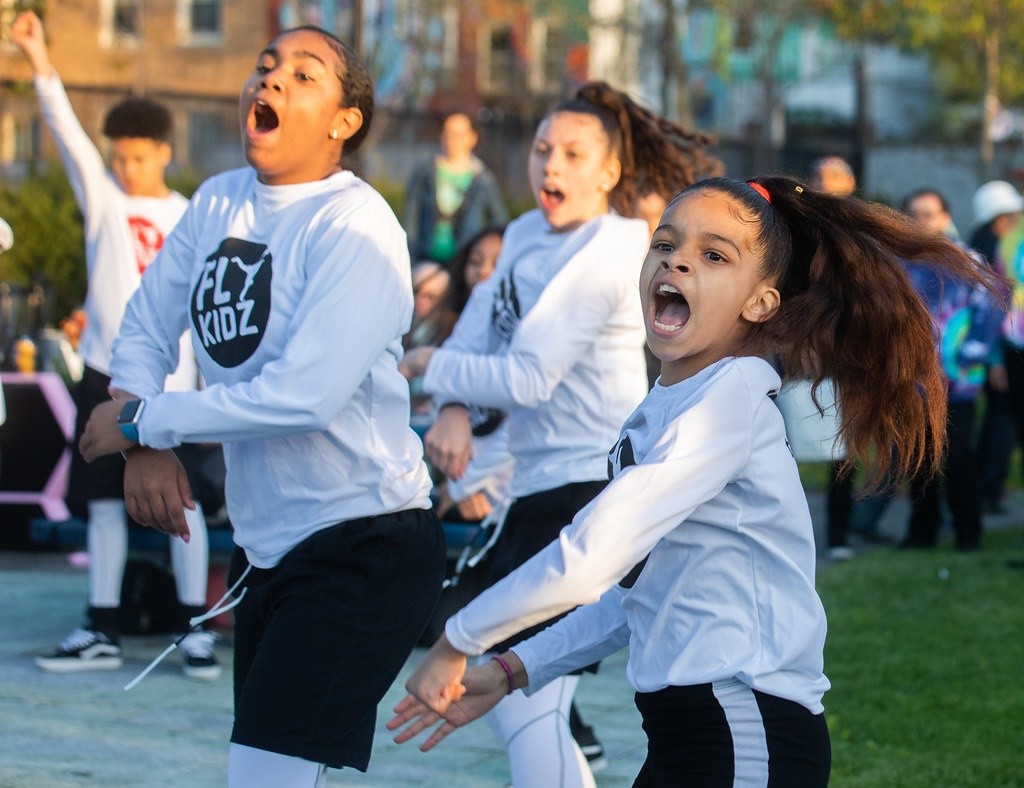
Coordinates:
[491,655,515,696]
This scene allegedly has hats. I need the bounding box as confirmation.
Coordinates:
[965,179,1024,245]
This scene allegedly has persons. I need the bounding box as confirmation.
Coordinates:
[770,156,1024,561]
[1,215,86,429]
[79,26,449,788]
[8,8,224,680]
[384,109,670,646]
[387,176,1023,788]
[397,80,726,788]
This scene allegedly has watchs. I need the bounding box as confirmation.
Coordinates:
[116,399,146,443]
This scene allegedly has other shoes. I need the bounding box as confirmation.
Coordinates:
[825,531,852,558]
[856,529,897,547]
[573,727,610,773]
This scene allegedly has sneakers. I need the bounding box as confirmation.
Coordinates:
[174,630,221,680]
[34,623,124,671]
